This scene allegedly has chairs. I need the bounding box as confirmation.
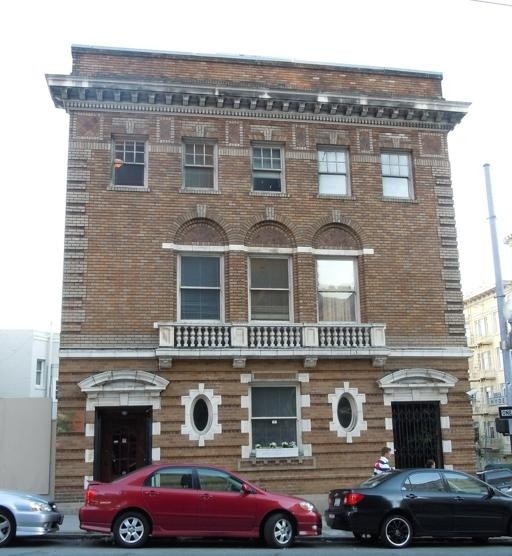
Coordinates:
[188,474,193,488]
[180,472,189,488]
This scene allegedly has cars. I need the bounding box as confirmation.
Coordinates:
[0,490,65,546]
[324,469,512,548]
[79,463,322,550]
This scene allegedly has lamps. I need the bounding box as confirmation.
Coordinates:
[114,158,127,169]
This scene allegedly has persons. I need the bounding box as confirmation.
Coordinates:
[374,446,391,475]
[426,459,436,469]
